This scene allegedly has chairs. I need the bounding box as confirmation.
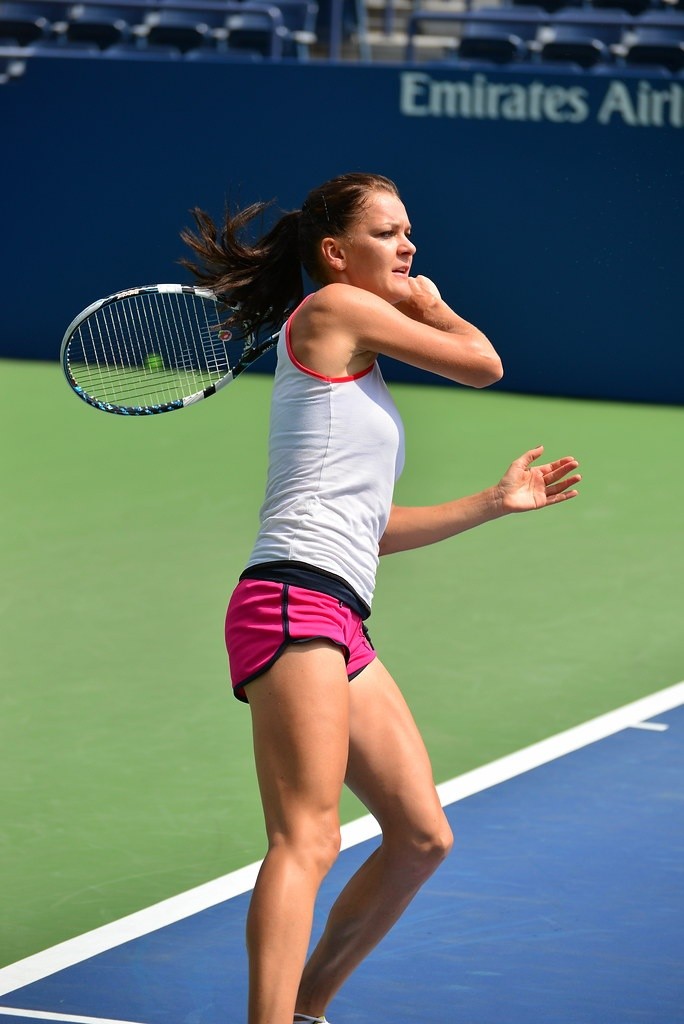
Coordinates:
[0,0,684,72]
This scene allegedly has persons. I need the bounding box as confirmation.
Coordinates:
[176,170,581,1024]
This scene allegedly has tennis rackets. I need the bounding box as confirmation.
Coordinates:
[58,280,283,417]
